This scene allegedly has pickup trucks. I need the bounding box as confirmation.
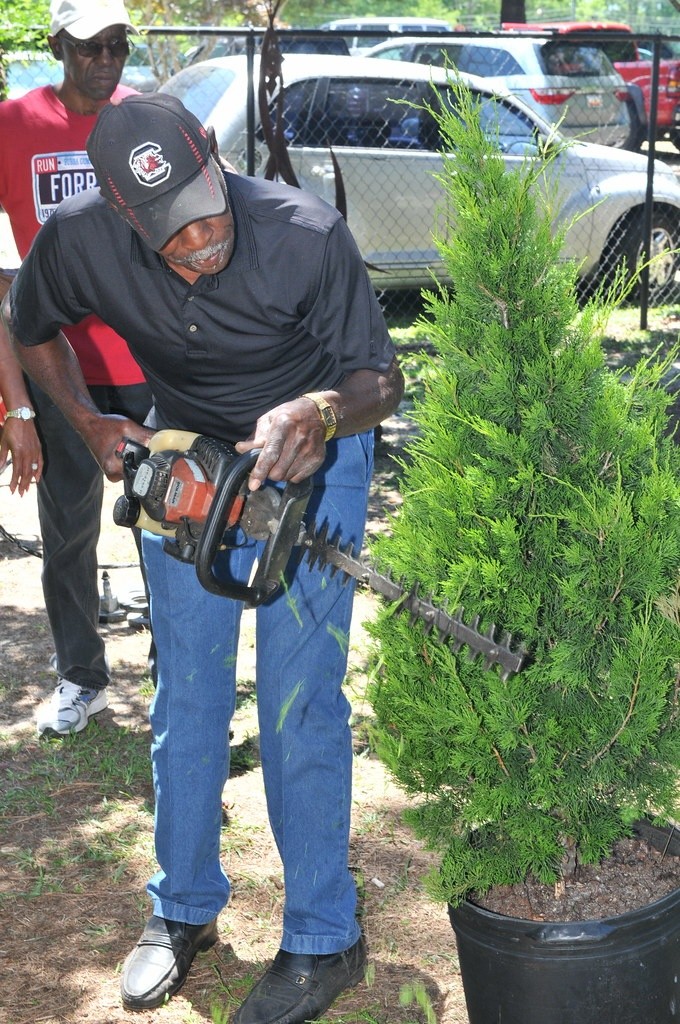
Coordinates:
[490,21,680,151]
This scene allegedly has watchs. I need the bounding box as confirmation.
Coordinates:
[6,407,36,420]
[302,392,338,441]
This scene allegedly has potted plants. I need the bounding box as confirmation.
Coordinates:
[343,48,680,1024]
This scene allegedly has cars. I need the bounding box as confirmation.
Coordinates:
[159,52,680,306]
[120,43,183,94]
[1,51,66,101]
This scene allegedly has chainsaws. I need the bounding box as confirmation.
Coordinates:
[113,428,524,684]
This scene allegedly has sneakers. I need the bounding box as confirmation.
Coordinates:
[37,677,107,740]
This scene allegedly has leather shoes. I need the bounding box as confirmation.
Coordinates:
[233,936,368,1024]
[120,915,218,1009]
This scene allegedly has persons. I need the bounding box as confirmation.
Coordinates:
[0,0,159,734]
[0,277,46,496]
[5,92,406,1024]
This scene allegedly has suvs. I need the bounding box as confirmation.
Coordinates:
[361,35,630,148]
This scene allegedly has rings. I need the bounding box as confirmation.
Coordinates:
[31,463,38,470]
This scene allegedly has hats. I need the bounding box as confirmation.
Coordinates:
[48,0,139,40]
[85,91,226,253]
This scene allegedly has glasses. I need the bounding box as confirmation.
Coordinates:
[59,35,128,57]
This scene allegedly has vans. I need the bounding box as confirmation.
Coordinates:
[321,18,455,54]
[143,31,351,93]
